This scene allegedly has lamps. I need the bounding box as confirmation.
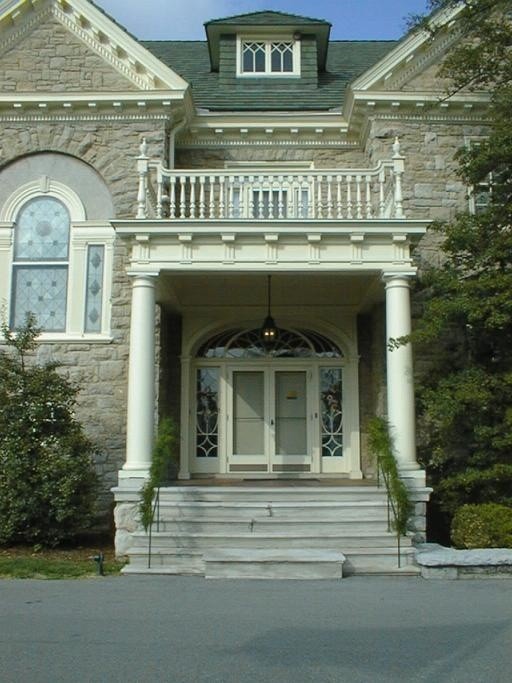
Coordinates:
[262,274,277,346]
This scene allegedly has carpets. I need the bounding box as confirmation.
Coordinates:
[244,477,319,482]
